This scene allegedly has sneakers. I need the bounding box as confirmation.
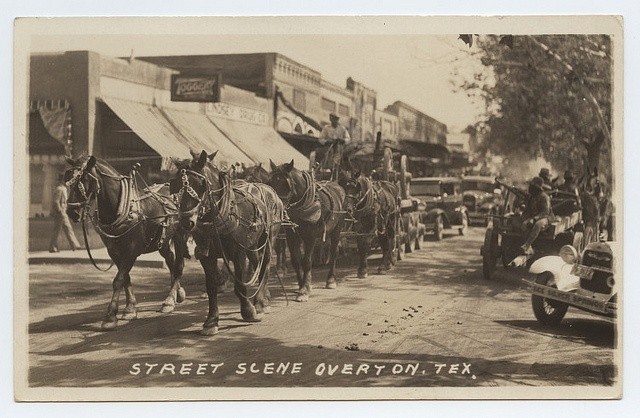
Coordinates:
[524,246,535,254]
[521,242,530,251]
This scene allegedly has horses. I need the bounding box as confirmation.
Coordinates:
[189,148,231,294]
[267,157,346,302]
[338,169,402,280]
[63,149,191,331]
[238,161,288,279]
[169,149,285,336]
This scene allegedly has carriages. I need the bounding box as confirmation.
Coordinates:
[480,166,615,279]
[64,130,427,336]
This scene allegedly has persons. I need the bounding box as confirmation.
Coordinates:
[319,112,352,145]
[537,169,555,189]
[519,176,555,255]
[558,170,583,209]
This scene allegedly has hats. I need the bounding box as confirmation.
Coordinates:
[564,170,573,179]
[539,168,551,176]
[527,177,544,191]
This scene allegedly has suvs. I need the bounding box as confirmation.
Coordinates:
[461,176,504,227]
[520,231,615,323]
[410,177,470,240]
